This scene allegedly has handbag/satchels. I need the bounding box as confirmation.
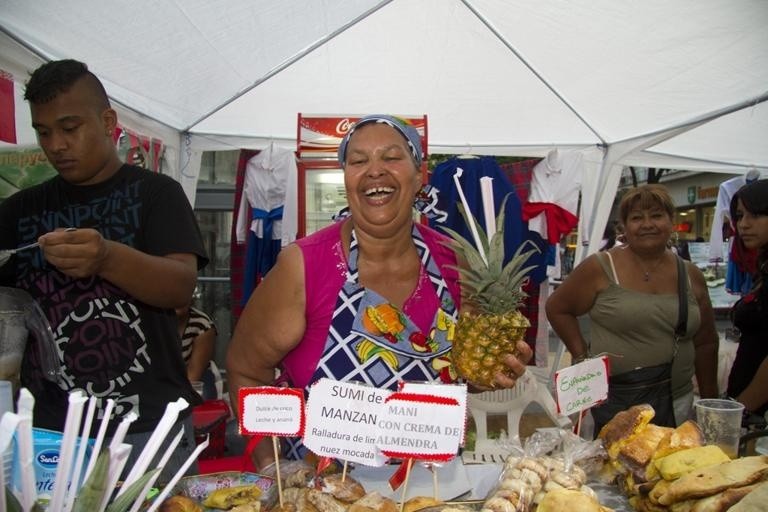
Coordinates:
[591,363,677,440]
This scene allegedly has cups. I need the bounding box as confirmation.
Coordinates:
[0,381,15,484]
[694,399,741,458]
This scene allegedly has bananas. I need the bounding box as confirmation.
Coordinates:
[358,340,398,369]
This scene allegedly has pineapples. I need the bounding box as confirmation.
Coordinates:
[450,309,531,391]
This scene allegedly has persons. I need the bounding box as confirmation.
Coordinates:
[175,301,219,401]
[224,114,532,484]
[544,184,719,443]
[0,60,210,491]
[727,179,768,427]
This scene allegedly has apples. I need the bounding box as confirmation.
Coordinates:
[432,356,452,370]
[440,366,458,383]
[409,331,426,346]
[411,342,431,353]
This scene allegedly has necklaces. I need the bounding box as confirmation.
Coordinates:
[634,253,662,281]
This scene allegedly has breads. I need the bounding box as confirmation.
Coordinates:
[115,403,768,512]
